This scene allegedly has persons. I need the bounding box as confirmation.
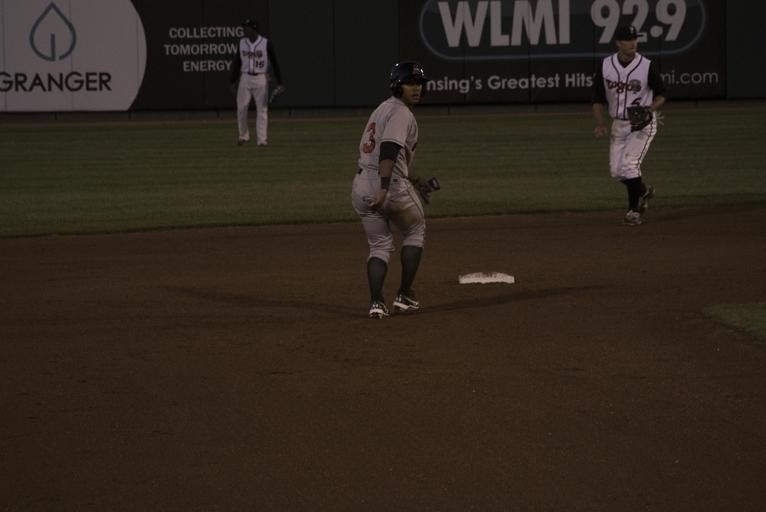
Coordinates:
[587,22,667,227]
[347,58,443,318]
[232,13,286,145]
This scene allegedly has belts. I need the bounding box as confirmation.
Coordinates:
[248,72,258,76]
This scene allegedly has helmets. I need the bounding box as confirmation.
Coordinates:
[389,62,430,98]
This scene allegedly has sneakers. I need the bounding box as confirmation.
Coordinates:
[368,299,391,318]
[392,294,420,312]
[256,139,268,147]
[637,184,656,214]
[622,208,648,226]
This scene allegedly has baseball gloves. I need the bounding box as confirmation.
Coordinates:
[411,176,440,204]
[268,79,286,105]
[627,106,653,132]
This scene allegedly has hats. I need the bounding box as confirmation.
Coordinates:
[614,24,644,41]
[240,18,258,29]
